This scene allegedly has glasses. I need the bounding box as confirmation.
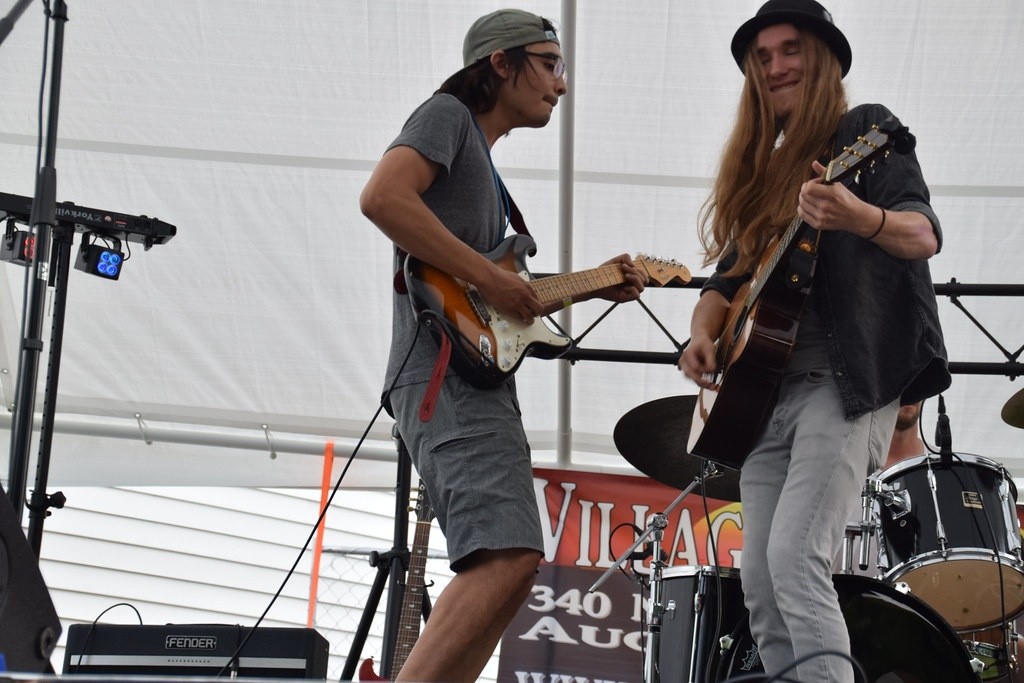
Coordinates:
[513,47,567,79]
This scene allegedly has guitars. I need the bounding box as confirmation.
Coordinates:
[358,477,436,683]
[685,113,916,473]
[404,232,694,393]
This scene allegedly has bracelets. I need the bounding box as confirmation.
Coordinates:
[868,205,886,240]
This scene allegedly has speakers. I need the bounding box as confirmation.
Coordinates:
[0,484,63,683]
[61,623,330,683]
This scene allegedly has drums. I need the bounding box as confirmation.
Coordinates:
[874,448,1024,635]
[716,572,986,682]
[652,564,743,683]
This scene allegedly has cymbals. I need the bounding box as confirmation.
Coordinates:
[1000,386,1024,430]
[611,392,743,503]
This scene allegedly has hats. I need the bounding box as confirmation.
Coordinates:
[440,9,561,87]
[730,0,852,79]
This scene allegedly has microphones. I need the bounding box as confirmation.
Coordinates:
[935,395,946,447]
[632,524,668,562]
[229,655,240,680]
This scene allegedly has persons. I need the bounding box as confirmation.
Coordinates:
[682,0,952,683]
[360,10,649,683]
[872,401,1024,683]
